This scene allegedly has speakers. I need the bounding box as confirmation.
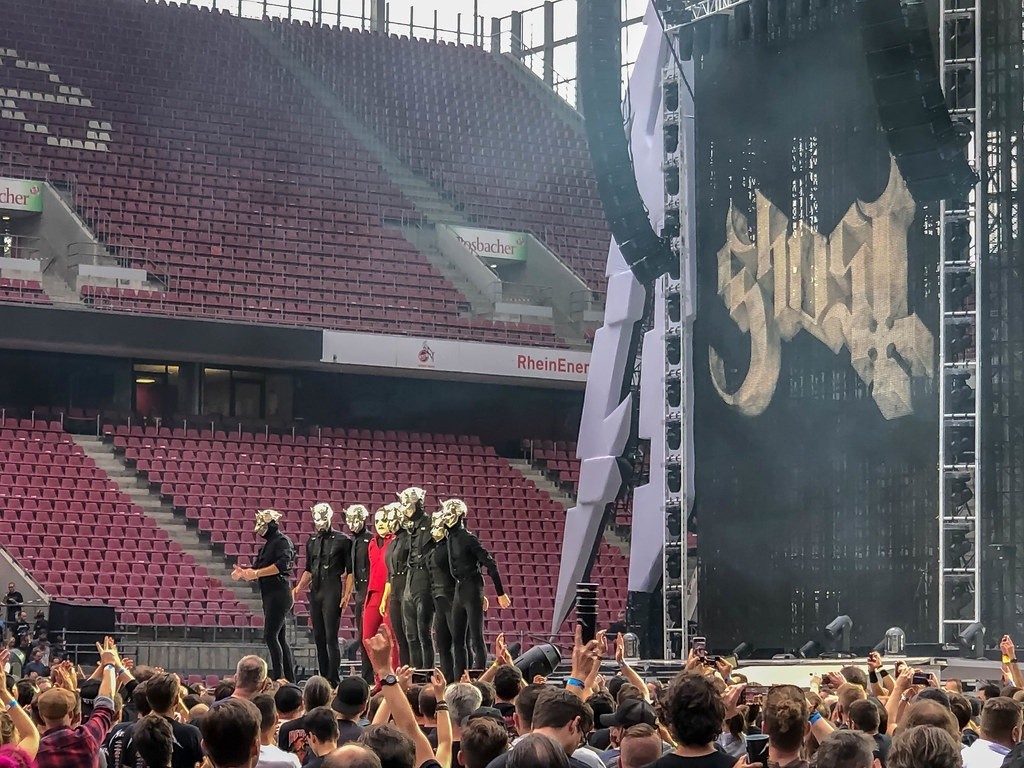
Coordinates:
[575,0,675,287]
[626,591,664,660]
[859,0,981,205]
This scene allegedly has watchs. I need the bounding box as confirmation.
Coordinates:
[380,674,399,685]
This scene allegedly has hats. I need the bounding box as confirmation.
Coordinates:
[274,683,302,713]
[912,687,951,712]
[331,676,370,716]
[605,675,632,703]
[585,690,619,730]
[6,674,15,693]
[38,688,76,719]
[461,706,508,733]
[600,699,659,730]
[79,680,101,715]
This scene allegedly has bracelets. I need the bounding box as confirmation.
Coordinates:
[7,700,18,709]
[877,665,890,678]
[869,670,878,684]
[567,678,585,690]
[103,662,115,668]
[620,662,625,667]
[255,570,259,579]
[117,668,126,674]
[1011,657,1017,663]
[810,711,821,725]
[492,662,498,668]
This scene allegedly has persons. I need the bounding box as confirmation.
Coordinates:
[0,580,54,663]
[340,504,375,688]
[0,624,1024,768]
[364,487,455,696]
[438,497,511,682]
[292,501,352,689]
[230,508,297,685]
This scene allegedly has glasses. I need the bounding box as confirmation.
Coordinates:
[578,721,587,748]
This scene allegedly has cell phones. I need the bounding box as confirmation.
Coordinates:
[411,669,437,685]
[914,673,933,681]
[693,637,706,658]
[912,677,928,684]
[895,663,902,677]
[741,686,771,706]
[605,633,617,641]
[869,653,876,662]
[696,656,720,671]
[822,674,833,685]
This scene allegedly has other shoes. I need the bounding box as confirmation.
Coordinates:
[370,683,382,697]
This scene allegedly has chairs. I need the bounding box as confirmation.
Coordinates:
[0,0,698,695]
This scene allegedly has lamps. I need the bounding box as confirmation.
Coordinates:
[732,639,758,660]
[824,615,857,658]
[798,640,820,659]
[958,622,988,660]
[511,642,562,684]
